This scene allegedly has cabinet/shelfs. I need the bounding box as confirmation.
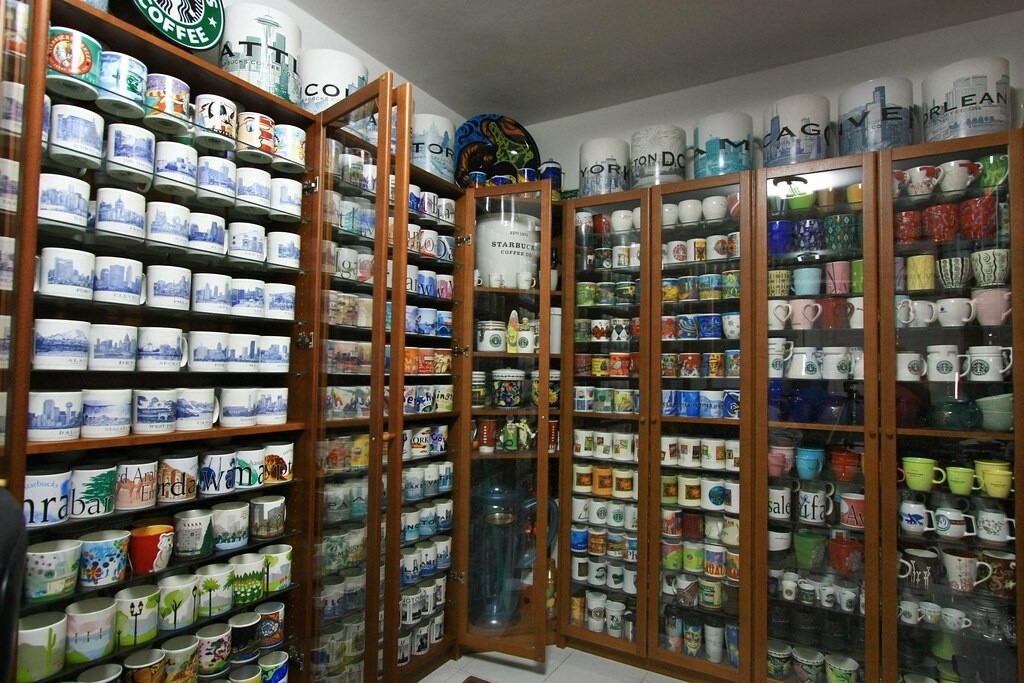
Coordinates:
[452,190,563,657]
[0,0,312,683]
[292,72,464,683]
[557,170,755,683]
[755,130,1024,683]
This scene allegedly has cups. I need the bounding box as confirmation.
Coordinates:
[477,321,506,352]
[573,205,641,416]
[662,192,741,422]
[516,272,536,289]
[0,0,308,683]
[489,275,506,289]
[471,416,560,454]
[492,369,526,409]
[488,176,513,187]
[765,446,882,683]
[531,369,561,409]
[469,481,558,630]
[312,139,456,683]
[896,458,1017,683]
[537,158,565,202]
[893,154,1015,434]
[474,270,483,286]
[539,270,558,291]
[468,172,486,188]
[517,331,539,353]
[760,181,873,426]
[570,423,639,641]
[471,372,486,408]
[550,308,562,354]
[517,168,540,200]
[659,434,742,672]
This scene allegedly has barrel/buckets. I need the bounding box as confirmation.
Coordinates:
[411,113,457,183]
[301,49,369,141]
[476,213,540,289]
[837,76,914,156]
[579,138,630,198]
[693,110,753,179]
[369,86,415,159]
[219,5,301,107]
[922,56,1012,142]
[763,93,837,167]
[630,126,686,190]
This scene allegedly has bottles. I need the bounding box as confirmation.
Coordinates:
[521,317,529,330]
[507,309,520,353]
[546,559,558,623]
